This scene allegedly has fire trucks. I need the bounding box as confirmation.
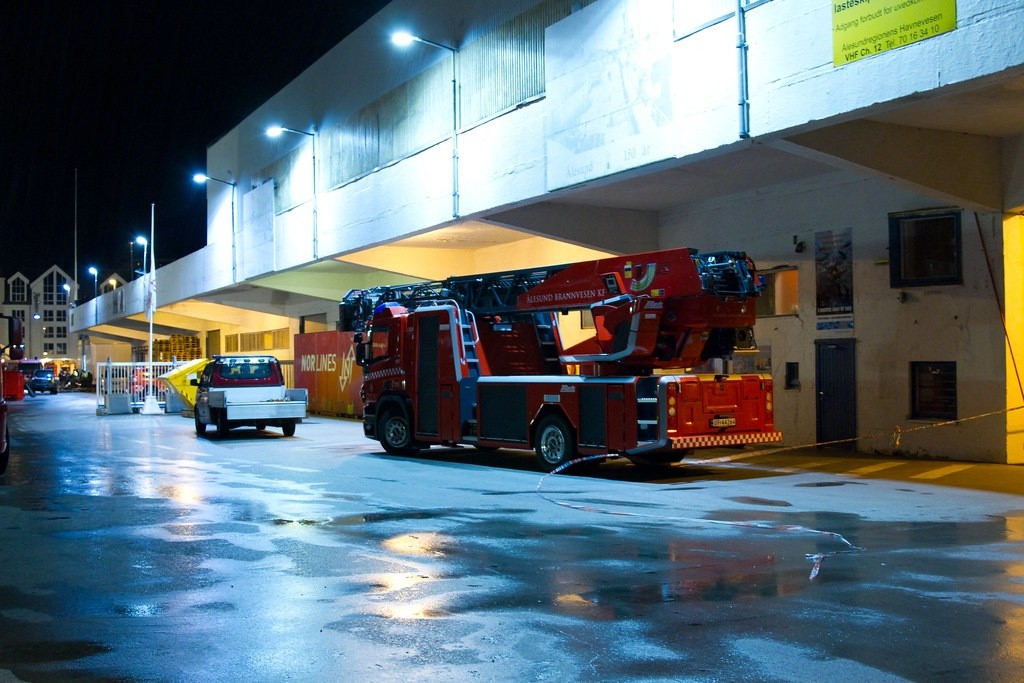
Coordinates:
[335,245,782,486]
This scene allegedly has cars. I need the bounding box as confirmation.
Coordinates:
[0,312,25,471]
[18,360,44,383]
[27,369,57,395]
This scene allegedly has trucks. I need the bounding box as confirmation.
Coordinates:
[190,353,309,436]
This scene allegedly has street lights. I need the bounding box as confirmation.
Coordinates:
[387,28,464,222]
[137,234,148,275]
[193,172,241,287]
[88,267,99,298]
[63,284,71,311]
[265,122,322,262]
